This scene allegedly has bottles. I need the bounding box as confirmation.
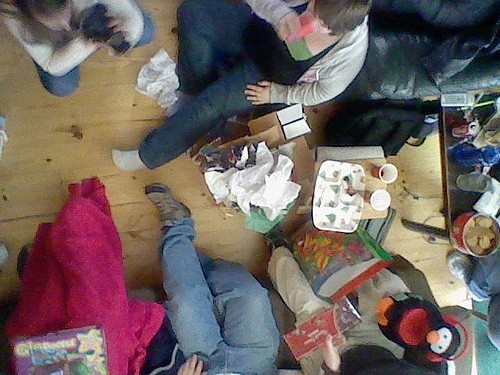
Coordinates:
[448,145,500,166]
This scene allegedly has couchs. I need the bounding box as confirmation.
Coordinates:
[337,0,500,120]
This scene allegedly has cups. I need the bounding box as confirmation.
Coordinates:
[371,164,398,184]
[456,173,492,193]
[364,189,391,211]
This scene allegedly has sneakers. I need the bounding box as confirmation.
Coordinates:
[447,250,485,302]
[263,221,293,255]
[145,183,191,222]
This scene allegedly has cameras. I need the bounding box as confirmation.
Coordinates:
[83,3,133,57]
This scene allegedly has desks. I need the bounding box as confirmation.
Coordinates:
[399,102,500,249]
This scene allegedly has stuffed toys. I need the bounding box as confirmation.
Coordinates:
[375,291,471,364]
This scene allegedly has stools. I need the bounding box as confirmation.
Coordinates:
[297,144,391,223]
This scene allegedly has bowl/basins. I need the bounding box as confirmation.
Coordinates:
[449,212,500,258]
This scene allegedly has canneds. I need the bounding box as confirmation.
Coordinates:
[453,121,479,138]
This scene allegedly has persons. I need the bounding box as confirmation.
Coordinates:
[4,175,166,375]
[109,0,370,171]
[145,182,282,375]
[264,221,448,375]
[0,0,154,96]
